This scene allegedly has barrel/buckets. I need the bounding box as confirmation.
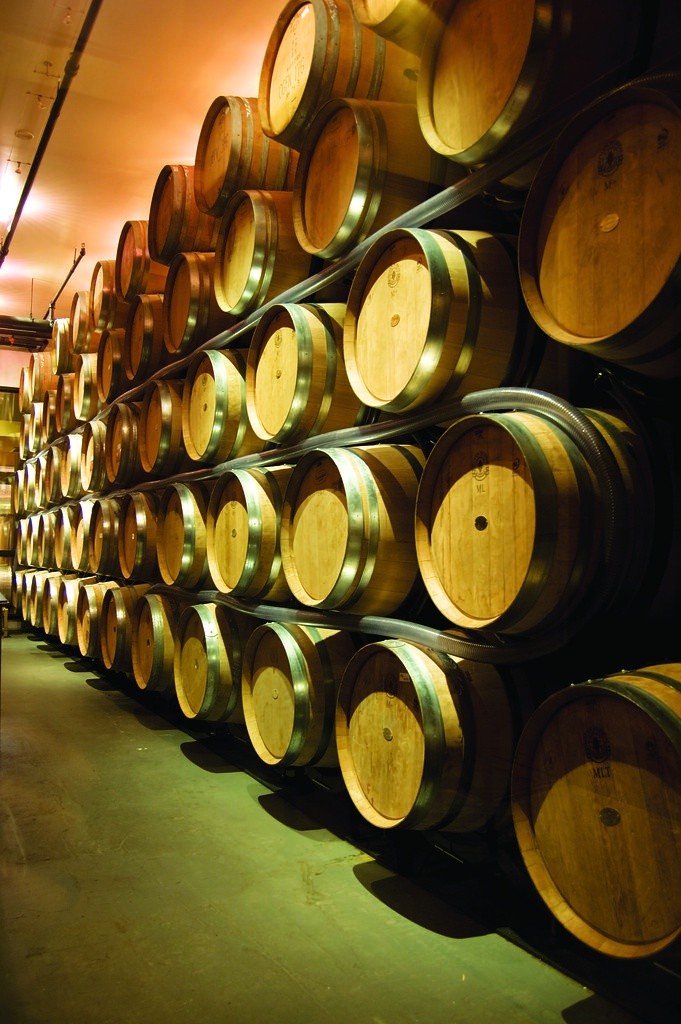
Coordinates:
[11,0,681,964]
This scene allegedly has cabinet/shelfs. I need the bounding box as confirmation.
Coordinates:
[15,62,681,979]
